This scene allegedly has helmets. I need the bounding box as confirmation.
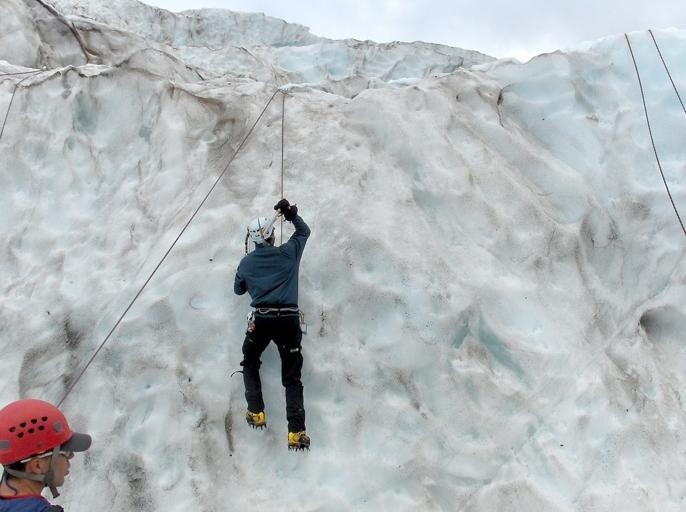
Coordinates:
[0,399,91,464]
[248,217,275,242]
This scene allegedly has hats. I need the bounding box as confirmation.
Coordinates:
[21,430,91,462]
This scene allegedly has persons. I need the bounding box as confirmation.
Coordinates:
[234,198,311,449]
[0,399,92,512]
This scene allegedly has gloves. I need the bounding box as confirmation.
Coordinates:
[274,198,298,221]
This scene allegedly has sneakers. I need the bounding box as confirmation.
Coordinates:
[287,430,311,452]
[245,408,266,431]
